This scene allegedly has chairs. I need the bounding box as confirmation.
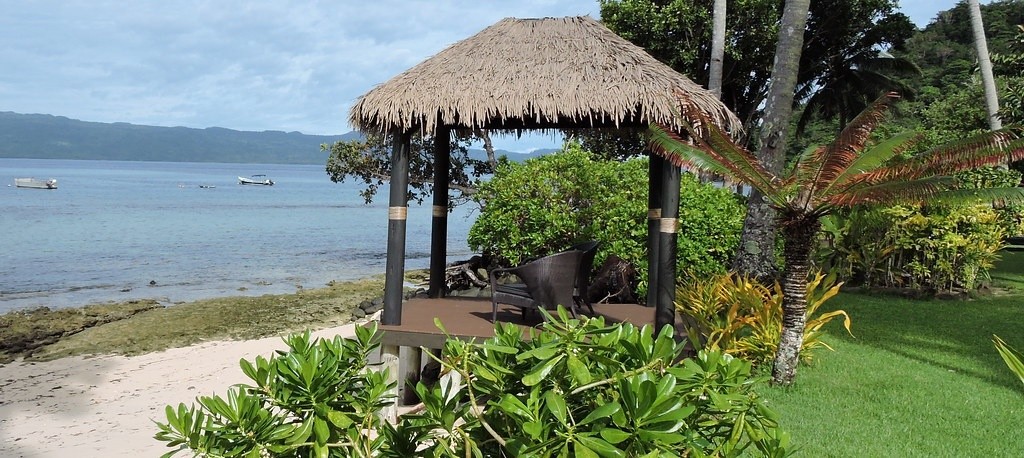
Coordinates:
[522,241,600,314]
[490,249,585,324]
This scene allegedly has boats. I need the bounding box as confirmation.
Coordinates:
[15,178,58,189]
[199,185,215,188]
[238,174,275,185]
